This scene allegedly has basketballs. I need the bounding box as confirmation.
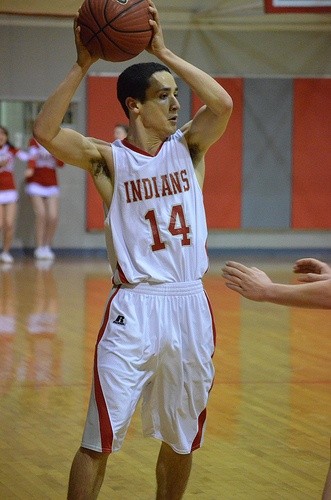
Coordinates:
[77,0,154,63]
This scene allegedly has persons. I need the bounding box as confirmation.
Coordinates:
[221,257,331,500]
[0,126,34,264]
[32,0,233,499]
[113,123,128,142]
[1,262,63,397]
[23,118,65,261]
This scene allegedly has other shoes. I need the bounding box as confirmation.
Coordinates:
[34,245,55,260]
[0,252,14,263]
